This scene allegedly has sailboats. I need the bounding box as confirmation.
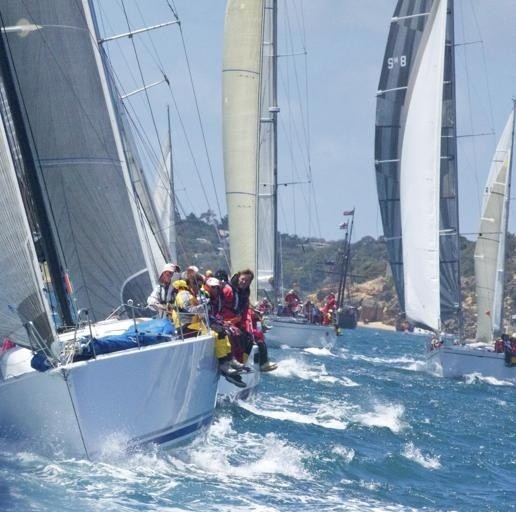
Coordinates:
[220,0,341,354]
[472,112,515,351]
[373,0,468,349]
[0,0,224,475]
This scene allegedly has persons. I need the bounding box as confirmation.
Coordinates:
[285,289,303,316]
[320,291,337,326]
[146,263,278,388]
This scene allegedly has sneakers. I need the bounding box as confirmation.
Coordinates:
[260,361,278,373]
[219,359,254,387]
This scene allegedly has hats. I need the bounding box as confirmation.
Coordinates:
[173,266,227,288]
